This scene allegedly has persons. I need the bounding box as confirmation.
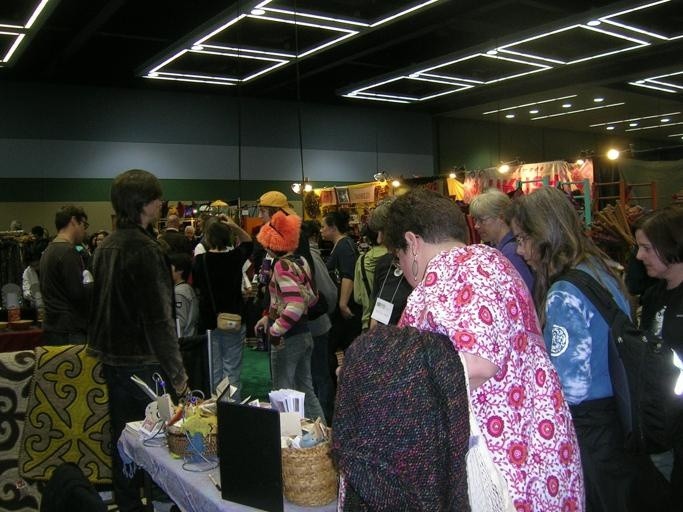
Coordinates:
[7,190,413,439]
[86,169,190,512]
[505,185,636,512]
[634,203,683,354]
[335,186,586,511]
[467,189,535,298]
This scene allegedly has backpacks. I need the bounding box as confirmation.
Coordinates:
[546,263,683,456]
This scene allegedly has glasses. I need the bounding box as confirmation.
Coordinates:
[517,229,536,248]
[392,235,420,269]
[81,220,90,229]
[472,214,499,225]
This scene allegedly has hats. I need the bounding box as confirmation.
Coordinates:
[255,210,302,253]
[256,190,297,215]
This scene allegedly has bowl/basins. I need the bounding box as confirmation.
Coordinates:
[10,319,32,331]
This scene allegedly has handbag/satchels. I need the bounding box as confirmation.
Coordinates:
[216,312,241,333]
[307,288,328,321]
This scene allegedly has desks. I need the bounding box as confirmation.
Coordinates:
[117,410,341,512]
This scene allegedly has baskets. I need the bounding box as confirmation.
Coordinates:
[280,427,340,508]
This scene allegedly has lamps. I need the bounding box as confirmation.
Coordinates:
[293,181,313,195]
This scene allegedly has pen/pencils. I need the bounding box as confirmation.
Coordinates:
[209,474,221,491]
